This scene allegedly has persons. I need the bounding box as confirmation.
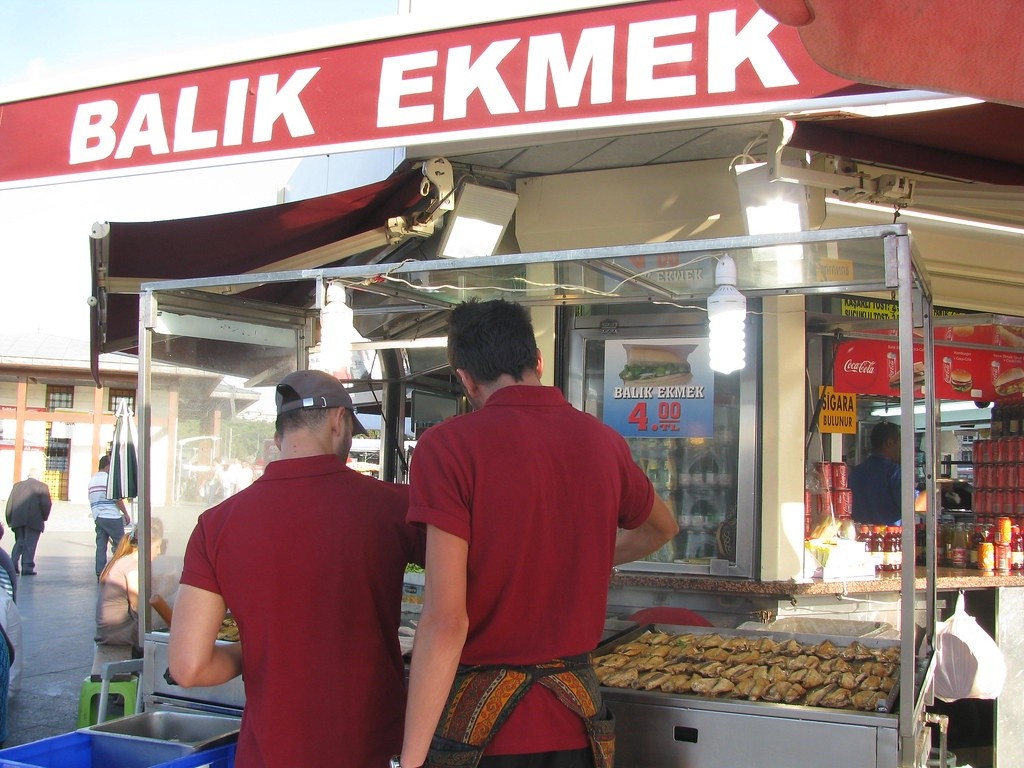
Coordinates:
[848,421,901,528]
[210,455,264,506]
[168,370,427,768]
[88,455,130,583]
[94,516,181,659]
[0,520,24,750]
[6,466,52,576]
[389,296,680,768]
[914,453,972,509]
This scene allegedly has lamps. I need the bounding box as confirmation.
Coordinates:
[321,251,747,376]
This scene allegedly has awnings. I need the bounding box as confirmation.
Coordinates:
[90,157,456,390]
[755,0,1024,112]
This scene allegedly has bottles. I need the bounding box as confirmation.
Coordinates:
[859,522,901,572]
[625,408,732,562]
[1010,528,1024,569]
[915,524,926,566]
[937,522,991,569]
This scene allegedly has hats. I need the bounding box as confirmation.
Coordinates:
[275,370,370,437]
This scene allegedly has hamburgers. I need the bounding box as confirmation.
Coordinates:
[619,348,692,390]
[888,361,926,391]
[994,367,1024,396]
[950,369,972,392]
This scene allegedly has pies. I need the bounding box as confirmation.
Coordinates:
[993,325,1024,347]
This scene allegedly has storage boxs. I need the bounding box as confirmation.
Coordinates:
[0,731,237,768]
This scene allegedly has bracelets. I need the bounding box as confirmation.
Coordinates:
[390,755,402,768]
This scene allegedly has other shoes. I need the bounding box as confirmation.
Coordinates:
[15,569,20,574]
[21,572,37,575]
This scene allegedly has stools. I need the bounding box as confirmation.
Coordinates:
[91,638,132,683]
[78,675,139,732]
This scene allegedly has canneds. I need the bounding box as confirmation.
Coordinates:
[991,361,1000,382]
[978,517,1011,571]
[943,357,952,384]
[971,437,1024,546]
[805,460,852,538]
[887,352,896,380]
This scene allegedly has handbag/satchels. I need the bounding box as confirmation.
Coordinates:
[931,592,1006,703]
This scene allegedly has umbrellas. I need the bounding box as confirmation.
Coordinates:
[105,398,138,530]
